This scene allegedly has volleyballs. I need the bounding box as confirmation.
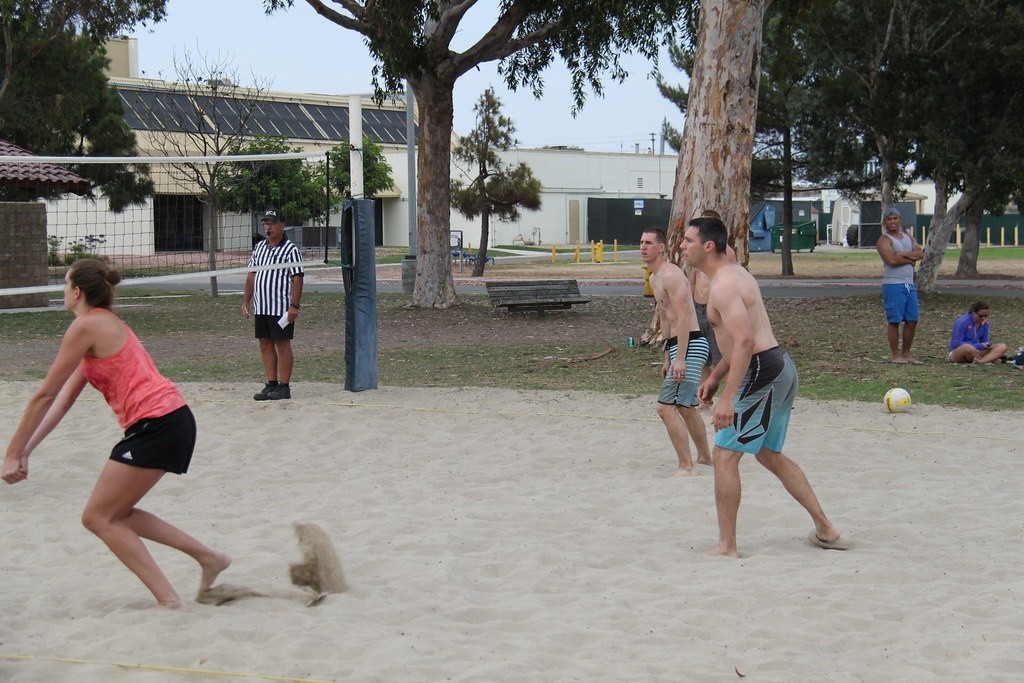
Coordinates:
[884,388,912,413]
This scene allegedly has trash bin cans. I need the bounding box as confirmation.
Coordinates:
[770,220,817,253]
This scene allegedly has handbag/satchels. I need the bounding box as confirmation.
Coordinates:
[1013,351,1024,364]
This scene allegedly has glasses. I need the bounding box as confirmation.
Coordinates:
[975,312,989,318]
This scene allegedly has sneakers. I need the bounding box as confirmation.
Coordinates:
[267,385,291,398]
[254,381,277,399]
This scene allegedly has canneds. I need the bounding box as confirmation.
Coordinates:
[627,336,634,348]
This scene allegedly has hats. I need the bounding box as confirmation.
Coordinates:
[261,210,285,222]
[884,208,900,219]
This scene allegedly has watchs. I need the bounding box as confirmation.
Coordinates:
[290,302,300,309]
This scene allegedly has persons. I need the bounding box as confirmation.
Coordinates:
[877,207,924,365]
[946,300,1007,366]
[0,258,232,613]
[639,210,849,558]
[241,209,304,400]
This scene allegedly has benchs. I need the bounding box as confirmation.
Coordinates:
[485,279,591,317]
